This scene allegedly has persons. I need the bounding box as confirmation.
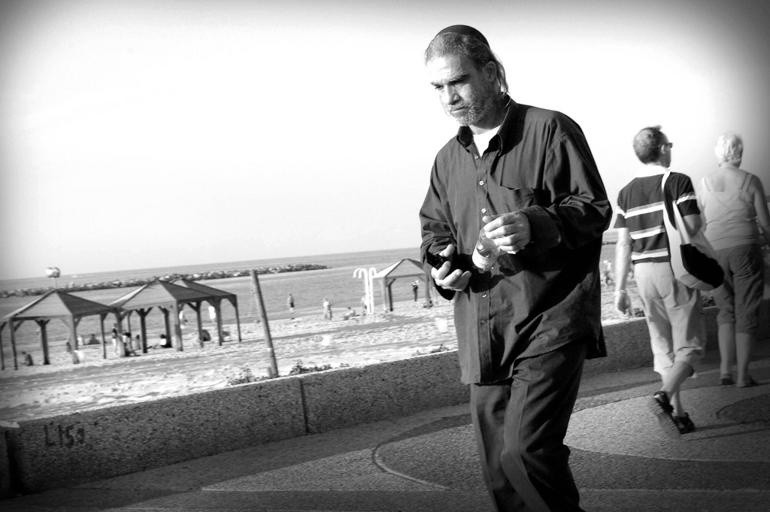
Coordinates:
[208,306,216,320]
[111,327,141,357]
[287,292,296,313]
[21,350,33,366]
[179,310,188,326]
[415,24,614,512]
[603,259,613,286]
[147,333,169,349]
[343,306,356,320]
[202,329,211,341]
[66,332,100,365]
[409,280,420,302]
[614,124,709,438]
[322,297,332,320]
[695,130,770,390]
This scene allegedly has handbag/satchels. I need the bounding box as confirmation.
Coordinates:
[662,199,726,291]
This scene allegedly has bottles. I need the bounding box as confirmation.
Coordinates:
[467,227,501,274]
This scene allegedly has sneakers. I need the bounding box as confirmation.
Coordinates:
[647,390,696,440]
[719,370,758,388]
